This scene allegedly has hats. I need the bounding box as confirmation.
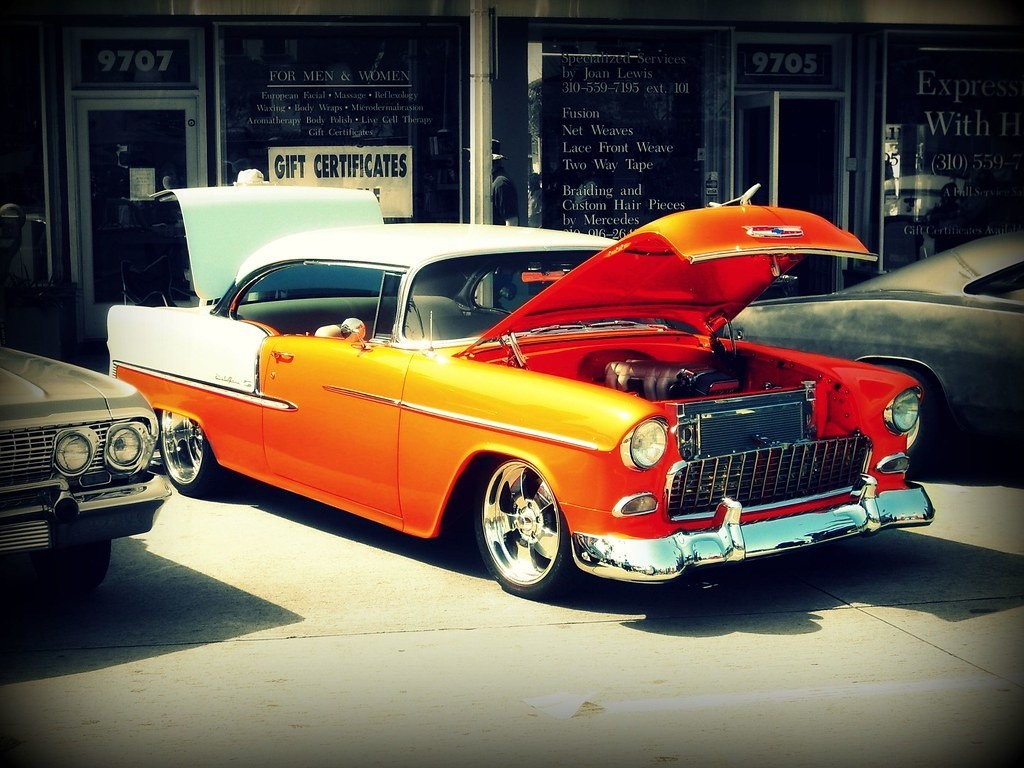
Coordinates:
[233,169,269,186]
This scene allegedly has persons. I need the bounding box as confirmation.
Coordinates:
[463,138,520,225]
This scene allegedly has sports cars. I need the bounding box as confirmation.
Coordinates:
[104,185,938,603]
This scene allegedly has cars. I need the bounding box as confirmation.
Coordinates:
[0,347,174,599]
[714,229,1023,482]
